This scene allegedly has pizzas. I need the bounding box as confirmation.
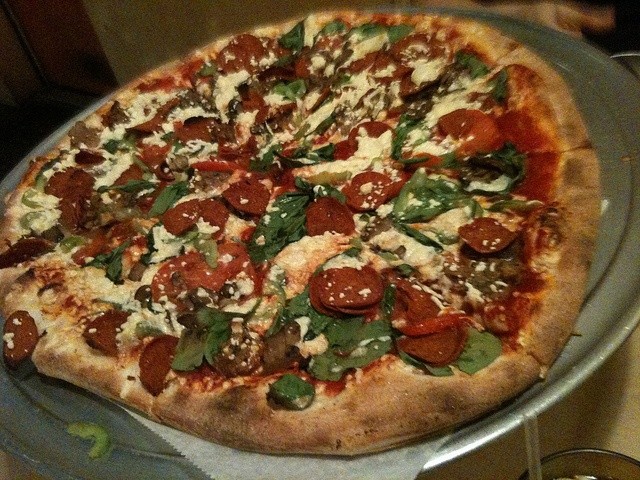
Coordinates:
[0,9,599,457]
